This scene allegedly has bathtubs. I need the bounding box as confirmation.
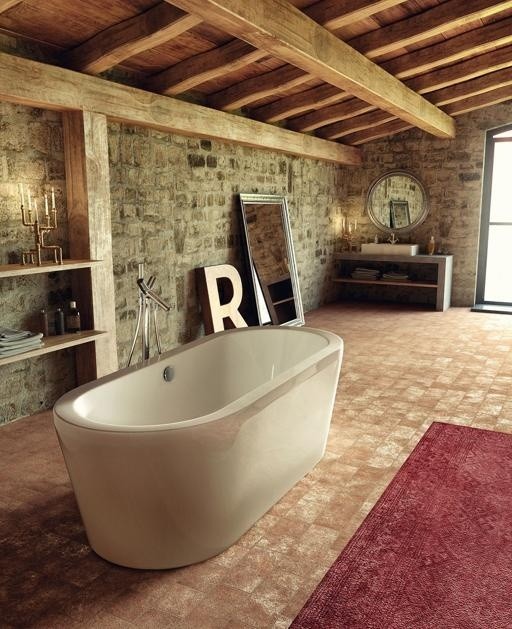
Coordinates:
[53,325,343,570]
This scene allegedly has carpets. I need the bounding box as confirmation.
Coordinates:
[287,418,511,629]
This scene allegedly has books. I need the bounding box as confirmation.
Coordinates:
[351,267,409,281]
[0,325,43,359]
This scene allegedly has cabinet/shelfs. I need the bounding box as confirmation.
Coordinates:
[0,258,118,364]
[331,250,455,314]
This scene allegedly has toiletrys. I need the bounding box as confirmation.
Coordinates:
[428,236,434,254]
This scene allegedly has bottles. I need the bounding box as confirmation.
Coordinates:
[39,301,81,337]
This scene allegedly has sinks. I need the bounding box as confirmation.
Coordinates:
[361,243,420,257]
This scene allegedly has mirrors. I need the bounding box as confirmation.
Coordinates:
[363,167,432,237]
[236,191,308,329]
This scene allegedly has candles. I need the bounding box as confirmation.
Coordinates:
[19,181,56,217]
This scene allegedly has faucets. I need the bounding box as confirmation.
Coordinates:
[146,288,171,312]
[387,232,399,244]
